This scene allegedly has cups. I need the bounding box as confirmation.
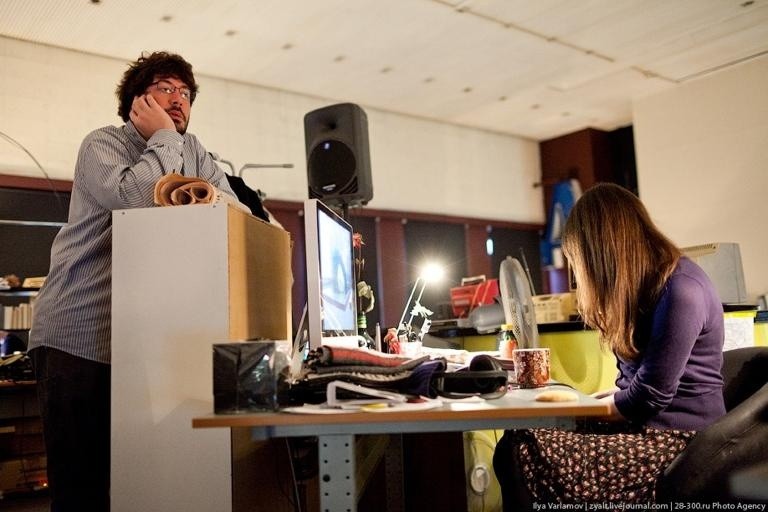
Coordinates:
[512,346,551,390]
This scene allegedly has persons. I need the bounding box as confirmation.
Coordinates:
[27,48,241,510]
[489,181,731,510]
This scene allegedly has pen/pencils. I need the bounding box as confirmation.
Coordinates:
[375,322,382,352]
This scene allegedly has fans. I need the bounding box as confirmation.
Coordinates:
[460,257,538,350]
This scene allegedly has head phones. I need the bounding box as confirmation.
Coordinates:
[428,355,509,399]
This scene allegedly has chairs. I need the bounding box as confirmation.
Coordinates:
[654,346,767,511]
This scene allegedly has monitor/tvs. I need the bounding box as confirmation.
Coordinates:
[294,199,358,358]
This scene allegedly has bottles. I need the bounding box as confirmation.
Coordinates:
[499,323,520,363]
[397,324,409,343]
[356,310,376,351]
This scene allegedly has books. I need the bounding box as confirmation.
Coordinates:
[0,274,49,331]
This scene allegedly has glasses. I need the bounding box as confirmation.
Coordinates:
[148,78,195,102]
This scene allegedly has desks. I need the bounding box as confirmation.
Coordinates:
[190,350,611,512]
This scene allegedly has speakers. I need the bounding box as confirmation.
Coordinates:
[304,103,373,209]
